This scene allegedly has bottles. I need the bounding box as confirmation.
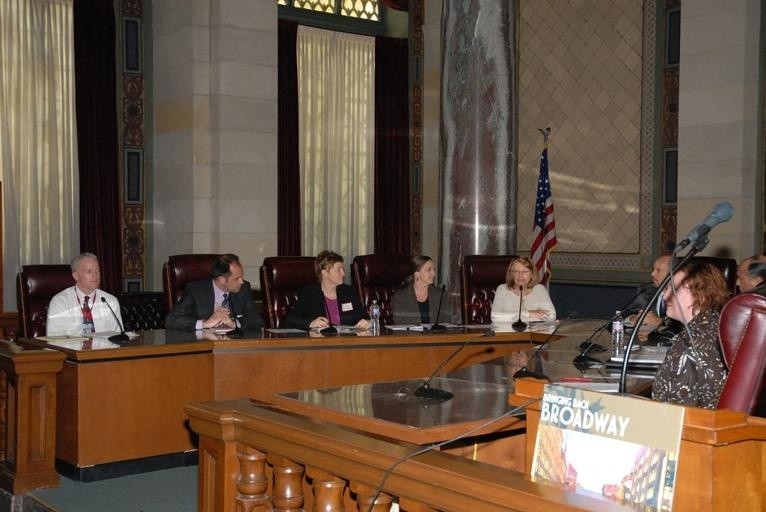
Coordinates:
[369,299,380,337]
[612,310,624,358]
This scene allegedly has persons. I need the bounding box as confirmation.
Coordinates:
[490,255,557,324]
[650,261,733,410]
[163,253,257,331]
[47,336,125,351]
[283,250,371,331]
[617,254,683,328]
[735,252,766,298]
[625,327,660,342]
[509,350,530,376]
[45,251,124,336]
[390,255,451,325]
[307,328,371,337]
[185,329,232,341]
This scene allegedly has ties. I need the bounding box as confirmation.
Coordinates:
[659,295,666,318]
[222,294,227,307]
[82,296,95,334]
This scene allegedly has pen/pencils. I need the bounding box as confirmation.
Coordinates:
[560,377,592,382]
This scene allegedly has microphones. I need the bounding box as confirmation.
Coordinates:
[319,290,338,335]
[571,304,639,367]
[430,284,448,332]
[223,292,245,339]
[576,309,639,352]
[620,287,648,310]
[511,285,527,330]
[414,330,496,402]
[674,200,735,255]
[100,296,130,342]
[692,236,710,251]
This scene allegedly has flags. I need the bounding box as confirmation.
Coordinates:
[529,143,560,294]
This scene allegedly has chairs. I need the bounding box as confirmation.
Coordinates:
[713,290,766,415]
[673,255,738,298]
[258,254,324,330]
[458,254,520,325]
[162,253,227,322]
[15,264,76,339]
[349,252,420,321]
